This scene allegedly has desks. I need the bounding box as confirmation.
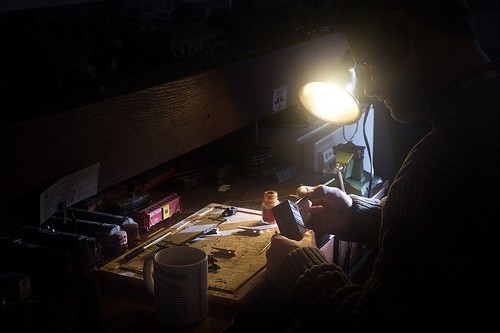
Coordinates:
[0,169,388,333]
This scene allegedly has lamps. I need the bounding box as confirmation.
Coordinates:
[296,47,409,199]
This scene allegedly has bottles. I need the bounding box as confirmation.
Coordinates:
[262,190,279,224]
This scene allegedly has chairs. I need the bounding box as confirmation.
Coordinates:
[0,225,104,333]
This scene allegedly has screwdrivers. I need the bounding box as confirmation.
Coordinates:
[119,230,172,265]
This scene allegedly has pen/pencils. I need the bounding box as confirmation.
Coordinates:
[293,177,336,207]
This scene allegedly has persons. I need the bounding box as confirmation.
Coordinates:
[264,0,500,333]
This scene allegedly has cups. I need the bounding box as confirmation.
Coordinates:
[143,246,208,326]
